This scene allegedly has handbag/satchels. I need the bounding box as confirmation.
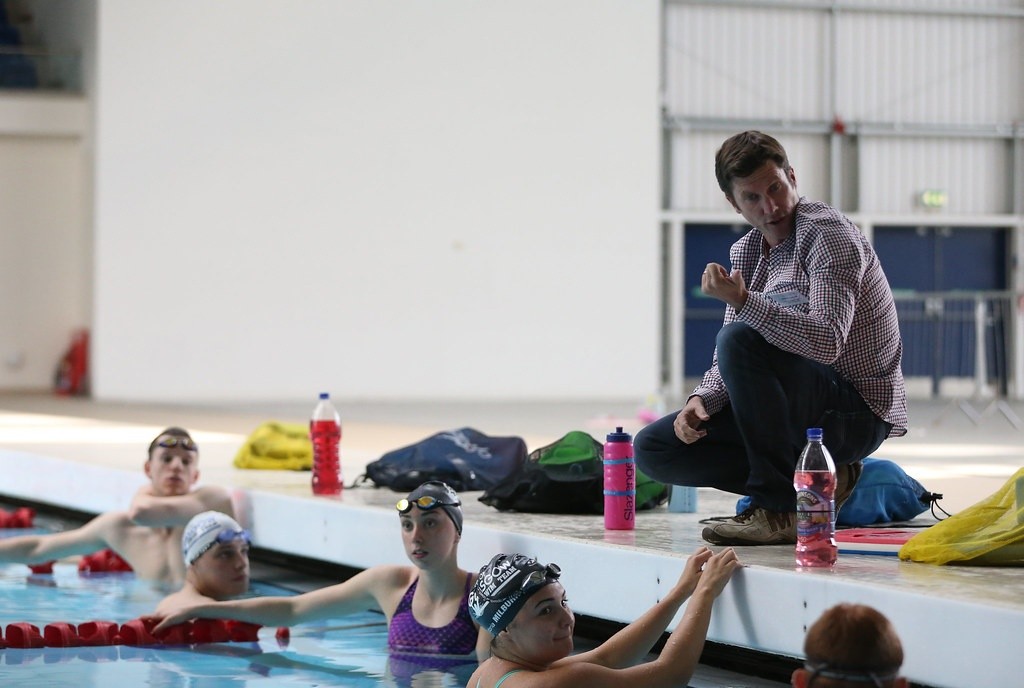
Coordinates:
[899,468,1024,565]
[342,428,527,491]
[735,457,951,527]
[478,431,670,516]
[234,421,315,471]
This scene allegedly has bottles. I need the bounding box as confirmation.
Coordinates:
[308,394,344,496]
[603,426,635,531]
[792,428,838,568]
[665,483,697,514]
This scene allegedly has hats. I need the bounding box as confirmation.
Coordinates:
[468,554,560,640]
[406,481,463,537]
[181,509,243,567]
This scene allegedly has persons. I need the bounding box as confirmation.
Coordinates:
[791,603,909,688]
[634,130,909,548]
[462,546,745,688]
[0,429,235,583]
[149,509,250,621]
[153,481,495,655]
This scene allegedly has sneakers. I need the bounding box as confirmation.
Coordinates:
[831,462,862,525]
[699,503,798,546]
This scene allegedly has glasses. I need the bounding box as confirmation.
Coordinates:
[395,495,442,514]
[159,438,197,451]
[520,562,561,593]
[216,530,252,546]
